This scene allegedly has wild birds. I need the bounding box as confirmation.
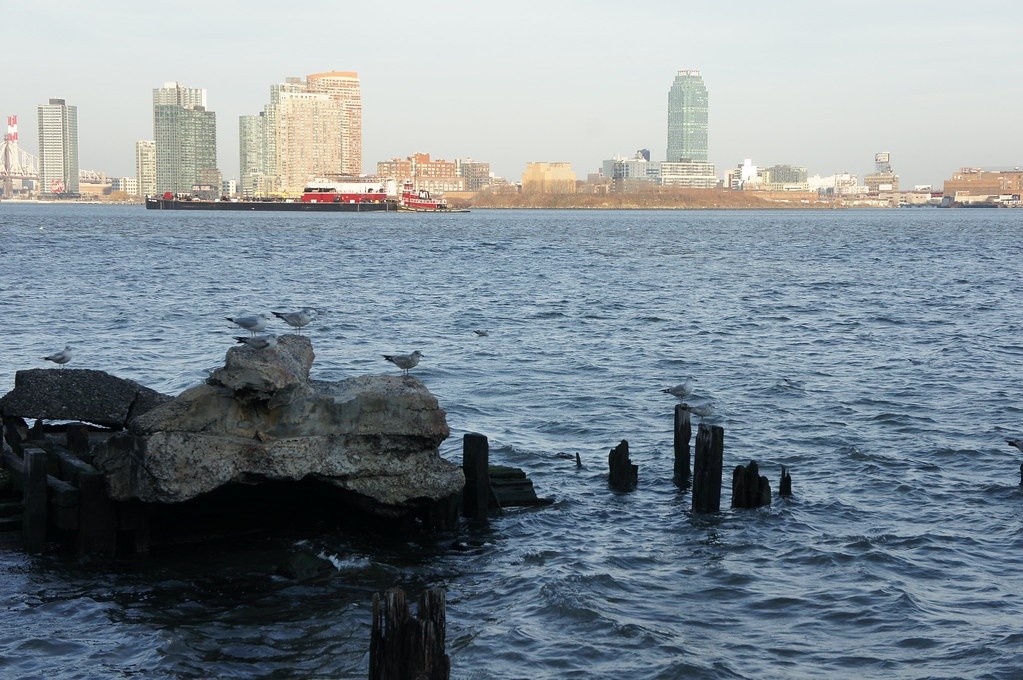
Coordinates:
[269,307,319,337]
[224,312,271,337]
[379,350,425,375]
[43,345,73,370]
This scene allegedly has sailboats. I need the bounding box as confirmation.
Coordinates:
[301,131,398,203]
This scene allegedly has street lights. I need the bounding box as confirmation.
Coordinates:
[174,182,177,197]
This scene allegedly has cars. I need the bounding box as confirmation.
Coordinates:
[187,196,192,201]
[193,198,200,202]
[220,195,229,201]
[243,196,275,202]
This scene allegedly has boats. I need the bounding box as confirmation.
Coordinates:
[899,203,930,208]
[402,180,447,209]
[930,202,998,208]
